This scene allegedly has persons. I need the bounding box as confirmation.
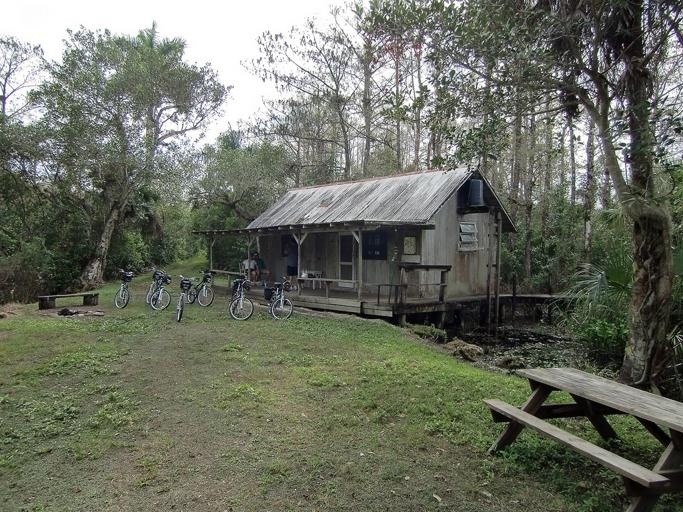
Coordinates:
[284,236,298,290]
[243,259,259,281]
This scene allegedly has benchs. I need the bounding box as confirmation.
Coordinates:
[35,290,98,309]
[483,399,670,511]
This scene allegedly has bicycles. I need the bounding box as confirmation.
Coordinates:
[173,274,196,323]
[268,276,296,323]
[144,265,173,311]
[188,269,216,308]
[113,269,135,310]
[226,269,257,324]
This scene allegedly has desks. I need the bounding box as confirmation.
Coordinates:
[299,270,323,290]
[483,354,682,512]
